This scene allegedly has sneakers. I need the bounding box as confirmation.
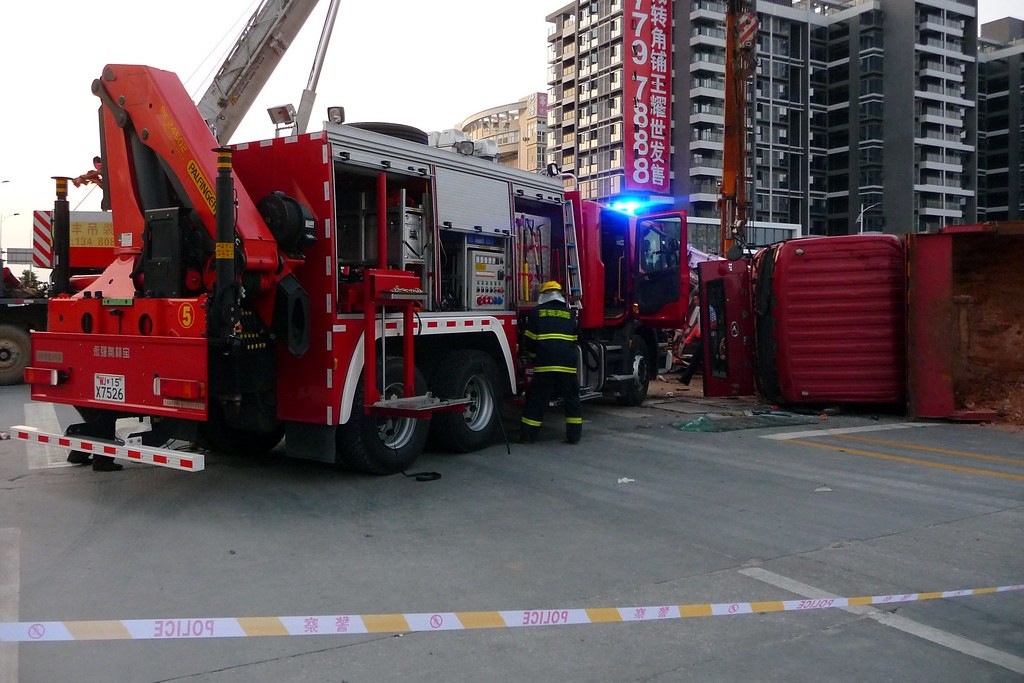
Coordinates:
[68,455,93,466]
[91,459,122,471]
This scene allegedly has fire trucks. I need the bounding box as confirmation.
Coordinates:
[31,1,697,476]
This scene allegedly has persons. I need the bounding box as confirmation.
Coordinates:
[507,281,583,445]
[675,291,718,386]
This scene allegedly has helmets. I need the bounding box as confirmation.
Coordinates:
[538,280,566,302]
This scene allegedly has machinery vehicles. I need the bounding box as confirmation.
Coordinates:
[1,1,342,386]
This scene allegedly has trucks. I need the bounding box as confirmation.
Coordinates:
[696,221,1024,423]
